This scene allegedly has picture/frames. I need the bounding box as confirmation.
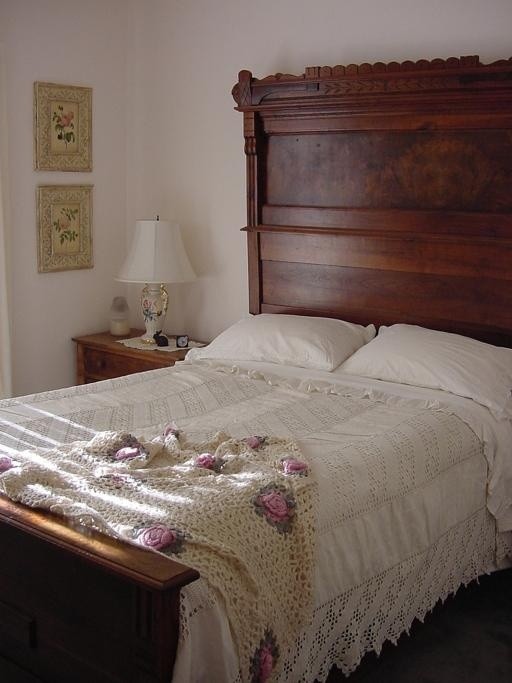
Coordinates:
[33,80,93,173]
[34,183,96,274]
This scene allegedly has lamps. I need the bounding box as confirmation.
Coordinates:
[113,214,197,344]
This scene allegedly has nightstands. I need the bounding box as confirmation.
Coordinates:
[67,327,209,385]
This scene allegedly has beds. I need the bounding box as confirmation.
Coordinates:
[0,53,511,682]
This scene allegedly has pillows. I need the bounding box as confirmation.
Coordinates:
[344,322,512,421]
[199,313,377,373]
